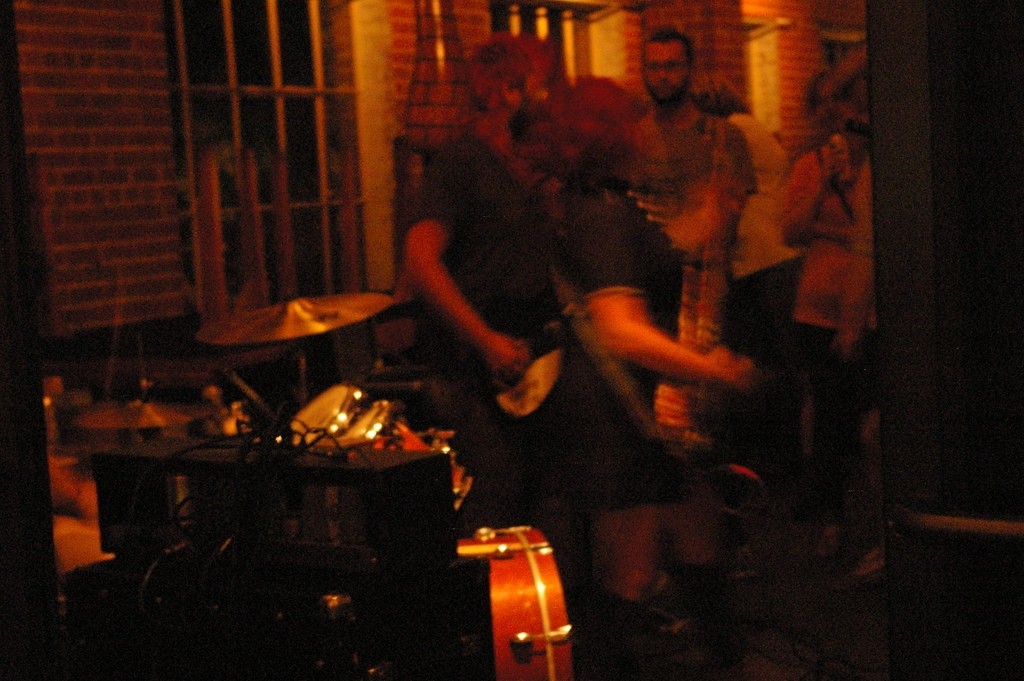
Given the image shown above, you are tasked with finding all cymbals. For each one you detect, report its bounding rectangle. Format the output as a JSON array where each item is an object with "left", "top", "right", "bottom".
[
  {"left": 74, "top": 402, "right": 206, "bottom": 434},
  {"left": 194, "top": 292, "right": 393, "bottom": 346}
]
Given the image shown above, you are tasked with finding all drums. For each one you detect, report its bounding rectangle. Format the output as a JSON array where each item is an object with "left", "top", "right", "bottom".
[
  {"left": 335, "top": 398, "right": 475, "bottom": 514},
  {"left": 453, "top": 524, "right": 575, "bottom": 681},
  {"left": 285, "top": 385, "right": 372, "bottom": 450}
]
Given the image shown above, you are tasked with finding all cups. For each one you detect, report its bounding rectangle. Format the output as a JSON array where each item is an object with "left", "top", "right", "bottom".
[{"left": 830, "top": 130, "right": 852, "bottom": 189}]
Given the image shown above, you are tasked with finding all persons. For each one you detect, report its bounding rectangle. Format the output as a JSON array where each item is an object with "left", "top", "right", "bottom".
[{"left": 402, "top": 20, "right": 874, "bottom": 681}]
